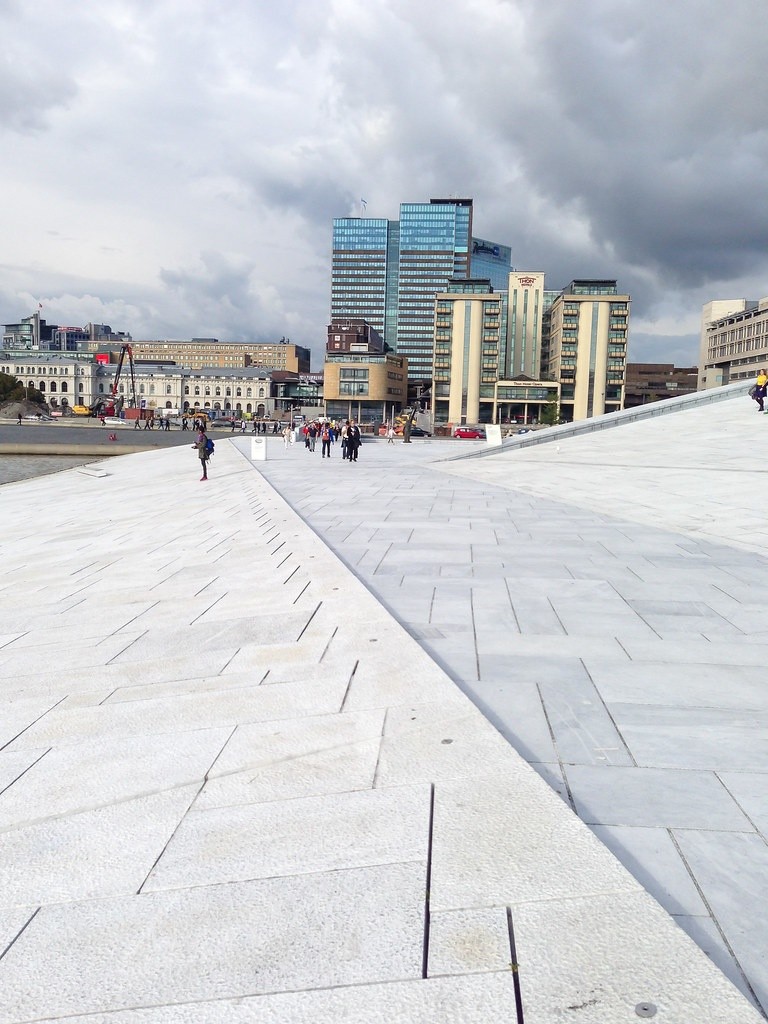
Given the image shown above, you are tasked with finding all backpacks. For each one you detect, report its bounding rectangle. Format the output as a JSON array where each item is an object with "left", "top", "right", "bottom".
[{"left": 204, "top": 438, "right": 214, "bottom": 455}]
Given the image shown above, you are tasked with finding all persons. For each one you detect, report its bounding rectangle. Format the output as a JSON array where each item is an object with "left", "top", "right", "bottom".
[
  {"left": 17, "top": 411, "right": 22, "bottom": 426},
  {"left": 231, "top": 417, "right": 362, "bottom": 462},
  {"left": 134, "top": 415, "right": 207, "bottom": 432},
  {"left": 387, "top": 427, "right": 397, "bottom": 444},
  {"left": 752, "top": 370, "right": 768, "bottom": 415},
  {"left": 191, "top": 426, "right": 209, "bottom": 481},
  {"left": 100, "top": 414, "right": 105, "bottom": 426}
]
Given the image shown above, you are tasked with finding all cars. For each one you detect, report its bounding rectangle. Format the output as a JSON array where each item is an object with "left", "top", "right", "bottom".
[
  {"left": 409, "top": 427, "right": 431, "bottom": 438},
  {"left": 454, "top": 428, "right": 486, "bottom": 439}
]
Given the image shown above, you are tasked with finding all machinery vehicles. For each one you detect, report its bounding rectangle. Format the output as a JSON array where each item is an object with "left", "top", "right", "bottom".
[
  {"left": 395, "top": 414, "right": 417, "bottom": 427},
  {"left": 71, "top": 343, "right": 137, "bottom": 418}
]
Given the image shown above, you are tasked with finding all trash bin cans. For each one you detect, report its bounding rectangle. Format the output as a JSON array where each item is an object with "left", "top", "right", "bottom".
[
  {"left": 250, "top": 437, "right": 267, "bottom": 461},
  {"left": 291, "top": 430, "right": 297, "bottom": 442}
]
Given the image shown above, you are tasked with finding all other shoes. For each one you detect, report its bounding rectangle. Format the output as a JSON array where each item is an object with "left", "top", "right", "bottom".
[
  {"left": 759, "top": 406, "right": 764, "bottom": 411},
  {"left": 200, "top": 477, "right": 208, "bottom": 481},
  {"left": 313, "top": 450, "right": 314, "bottom": 451}
]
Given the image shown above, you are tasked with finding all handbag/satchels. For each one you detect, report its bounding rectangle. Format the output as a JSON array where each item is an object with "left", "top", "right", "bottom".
[{"left": 322, "top": 436, "right": 330, "bottom": 441}]
